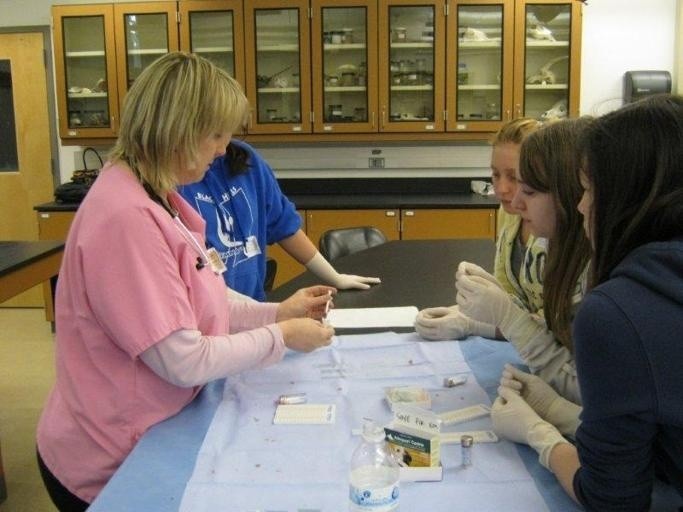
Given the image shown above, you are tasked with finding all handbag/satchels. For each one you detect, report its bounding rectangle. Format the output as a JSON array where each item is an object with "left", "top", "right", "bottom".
[{"left": 55, "top": 167, "right": 103, "bottom": 201}]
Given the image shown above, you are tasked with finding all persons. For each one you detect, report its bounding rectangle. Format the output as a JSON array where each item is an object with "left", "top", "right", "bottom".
[
  {"left": 454, "top": 115, "right": 592, "bottom": 407},
  {"left": 175, "top": 139, "right": 382, "bottom": 304},
  {"left": 33, "top": 53, "right": 336, "bottom": 511},
  {"left": 410, "top": 116, "right": 543, "bottom": 340},
  {"left": 490, "top": 94, "right": 683, "bottom": 509}
]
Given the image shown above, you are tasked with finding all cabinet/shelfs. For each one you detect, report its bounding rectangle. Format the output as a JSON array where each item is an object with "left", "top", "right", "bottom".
[
  {"left": 178, "top": 0, "right": 312, "bottom": 143},
  {"left": 306, "top": 208, "right": 498, "bottom": 253},
  {"left": 51, "top": 0, "right": 178, "bottom": 146},
  {"left": 445, "top": 0, "right": 583, "bottom": 142},
  {"left": 37, "top": 207, "right": 305, "bottom": 330},
  {"left": 310, "top": 0, "right": 445, "bottom": 142}
]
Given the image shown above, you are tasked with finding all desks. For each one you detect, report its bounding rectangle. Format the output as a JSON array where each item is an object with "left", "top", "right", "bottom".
[
  {"left": 83, "top": 237, "right": 683, "bottom": 512},
  {"left": 0, "top": 240, "right": 65, "bottom": 313}
]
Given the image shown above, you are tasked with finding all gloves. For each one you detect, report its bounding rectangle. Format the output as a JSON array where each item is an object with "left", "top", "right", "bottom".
[
  {"left": 414, "top": 261, "right": 583, "bottom": 472},
  {"left": 305, "top": 250, "right": 381, "bottom": 288}
]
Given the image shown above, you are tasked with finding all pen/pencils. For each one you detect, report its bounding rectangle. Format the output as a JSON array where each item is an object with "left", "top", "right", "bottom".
[{"left": 324, "top": 290, "right": 332, "bottom": 317}]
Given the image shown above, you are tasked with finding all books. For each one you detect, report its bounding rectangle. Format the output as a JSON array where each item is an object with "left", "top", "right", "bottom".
[{"left": 325, "top": 306, "right": 421, "bottom": 332}]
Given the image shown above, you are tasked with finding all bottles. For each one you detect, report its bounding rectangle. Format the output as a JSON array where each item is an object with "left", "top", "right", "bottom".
[
  {"left": 347, "top": 420, "right": 400, "bottom": 512},
  {"left": 268, "top": 27, "right": 498, "bottom": 121},
  {"left": 70, "top": 110, "right": 82, "bottom": 127},
  {"left": 460, "top": 435, "right": 475, "bottom": 468},
  {"left": 279, "top": 393, "right": 307, "bottom": 405},
  {"left": 368, "top": 150, "right": 385, "bottom": 168},
  {"left": 444, "top": 375, "right": 467, "bottom": 387}
]
